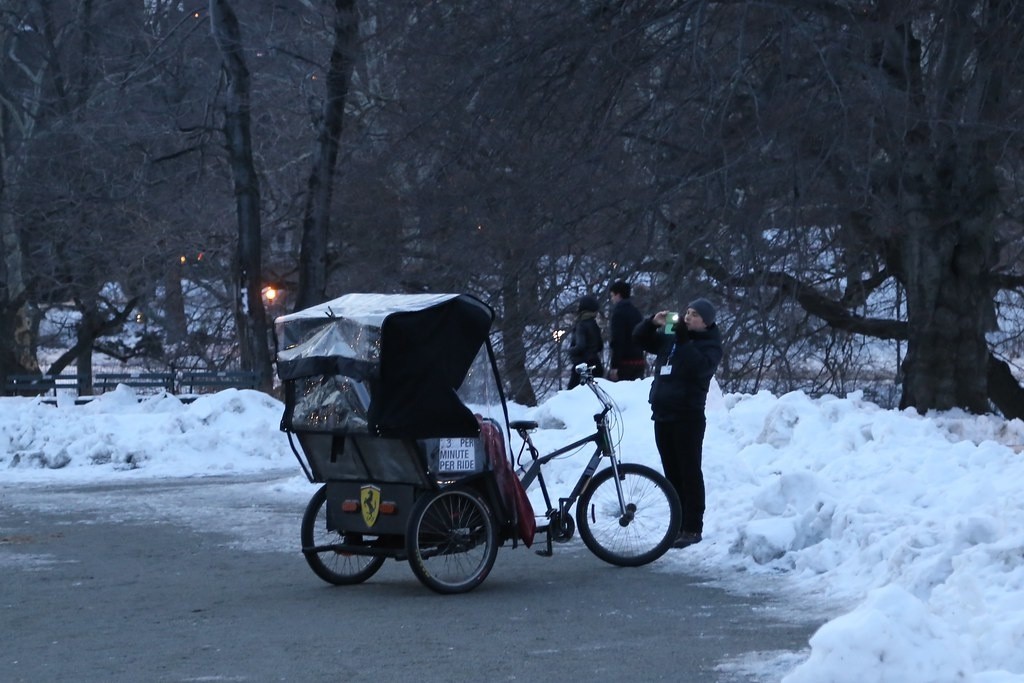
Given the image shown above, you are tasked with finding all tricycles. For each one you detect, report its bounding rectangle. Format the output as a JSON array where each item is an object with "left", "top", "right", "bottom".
[{"left": 271, "top": 291, "right": 683, "bottom": 594}]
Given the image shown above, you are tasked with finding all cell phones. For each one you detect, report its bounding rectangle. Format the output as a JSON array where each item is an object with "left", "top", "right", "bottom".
[{"left": 666, "top": 312, "right": 679, "bottom": 324}]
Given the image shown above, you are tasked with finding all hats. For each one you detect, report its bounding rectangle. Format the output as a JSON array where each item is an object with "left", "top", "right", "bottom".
[
  {"left": 610, "top": 282, "right": 631, "bottom": 299},
  {"left": 687, "top": 299, "right": 716, "bottom": 327},
  {"left": 579, "top": 296, "right": 600, "bottom": 313}
]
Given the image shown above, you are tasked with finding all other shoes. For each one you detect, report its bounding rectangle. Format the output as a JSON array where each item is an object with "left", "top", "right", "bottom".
[{"left": 673, "top": 532, "right": 702, "bottom": 548}]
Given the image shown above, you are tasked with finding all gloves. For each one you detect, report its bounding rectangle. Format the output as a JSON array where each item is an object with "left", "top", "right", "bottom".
[{"left": 671, "top": 316, "right": 688, "bottom": 341}]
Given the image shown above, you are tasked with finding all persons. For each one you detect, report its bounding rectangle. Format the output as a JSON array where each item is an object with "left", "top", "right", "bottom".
[
  {"left": 632, "top": 298, "right": 721, "bottom": 549},
  {"left": 606, "top": 281, "right": 645, "bottom": 383},
  {"left": 566, "top": 295, "right": 604, "bottom": 390}
]
